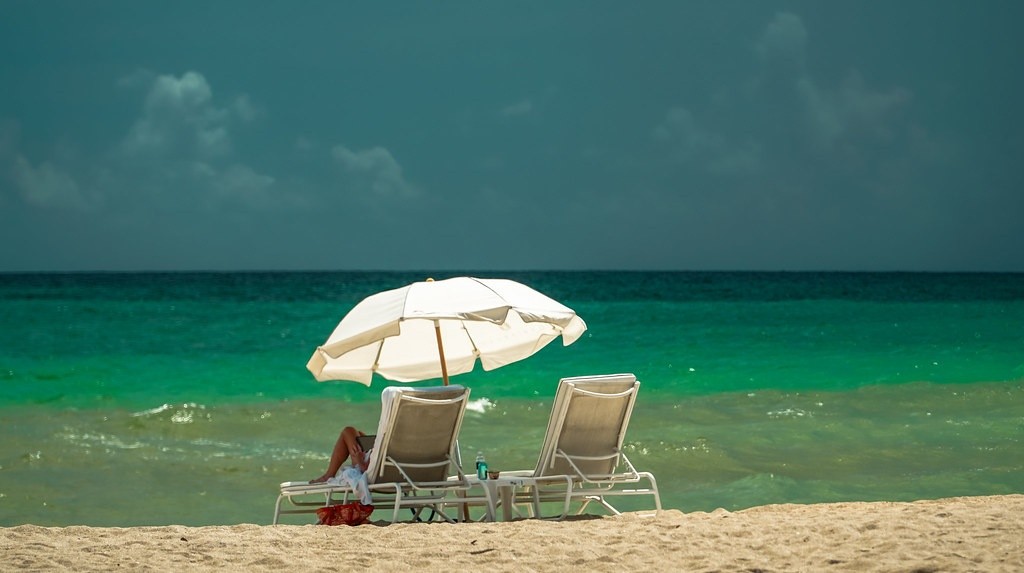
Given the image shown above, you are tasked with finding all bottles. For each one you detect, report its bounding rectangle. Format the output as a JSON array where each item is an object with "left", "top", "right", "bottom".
[
  {"left": 476, "top": 451, "right": 483, "bottom": 477},
  {"left": 478, "top": 456, "right": 488, "bottom": 480}
]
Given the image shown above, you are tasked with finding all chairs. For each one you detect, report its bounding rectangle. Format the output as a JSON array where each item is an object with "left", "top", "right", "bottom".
[
  {"left": 434, "top": 373, "right": 661, "bottom": 517},
  {"left": 273, "top": 383, "right": 497, "bottom": 526}
]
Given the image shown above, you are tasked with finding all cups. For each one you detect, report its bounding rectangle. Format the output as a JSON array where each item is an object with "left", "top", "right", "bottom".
[{"left": 489, "top": 471, "right": 500, "bottom": 479}]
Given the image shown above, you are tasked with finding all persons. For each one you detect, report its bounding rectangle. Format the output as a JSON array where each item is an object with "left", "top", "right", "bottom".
[{"left": 309, "top": 426, "right": 378, "bottom": 484}]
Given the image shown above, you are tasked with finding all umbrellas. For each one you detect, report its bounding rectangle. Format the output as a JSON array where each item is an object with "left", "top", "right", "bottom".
[{"left": 306, "top": 275, "right": 588, "bottom": 520}]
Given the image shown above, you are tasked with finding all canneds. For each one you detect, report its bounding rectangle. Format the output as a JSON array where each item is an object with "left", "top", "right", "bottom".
[{"left": 478, "top": 462, "right": 487, "bottom": 480}]
